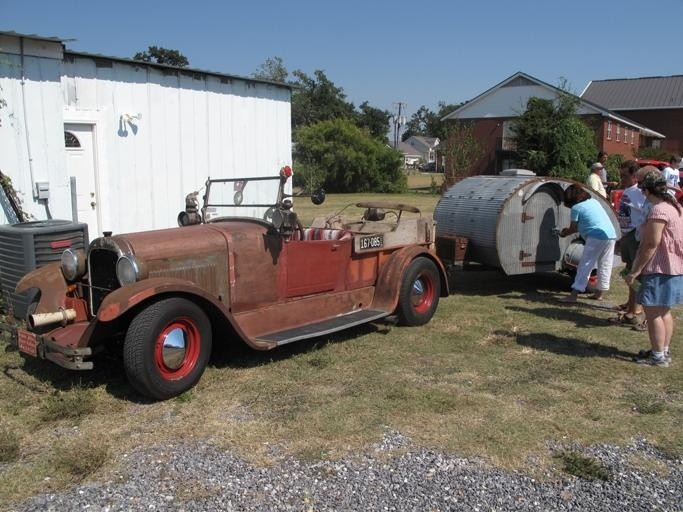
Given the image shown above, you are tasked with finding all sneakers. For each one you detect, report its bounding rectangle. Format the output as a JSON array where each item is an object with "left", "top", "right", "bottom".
[
  {"left": 632, "top": 321, "right": 649, "bottom": 333},
  {"left": 613, "top": 304, "right": 632, "bottom": 312},
  {"left": 609, "top": 313, "right": 639, "bottom": 328},
  {"left": 636, "top": 356, "right": 670, "bottom": 368},
  {"left": 640, "top": 347, "right": 674, "bottom": 364}
]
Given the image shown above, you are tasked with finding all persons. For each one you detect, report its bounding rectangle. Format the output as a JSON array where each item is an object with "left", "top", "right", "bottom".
[
  {"left": 624, "top": 170, "right": 683, "bottom": 366},
  {"left": 597, "top": 153, "right": 609, "bottom": 183},
  {"left": 607, "top": 166, "right": 663, "bottom": 330},
  {"left": 558, "top": 183, "right": 617, "bottom": 303},
  {"left": 614, "top": 160, "right": 647, "bottom": 312},
  {"left": 662, "top": 155, "right": 682, "bottom": 198},
  {"left": 585, "top": 163, "right": 618, "bottom": 201}
]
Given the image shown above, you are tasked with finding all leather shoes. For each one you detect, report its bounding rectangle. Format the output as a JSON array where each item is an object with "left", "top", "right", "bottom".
[
  {"left": 587, "top": 294, "right": 603, "bottom": 301},
  {"left": 556, "top": 296, "right": 579, "bottom": 303}
]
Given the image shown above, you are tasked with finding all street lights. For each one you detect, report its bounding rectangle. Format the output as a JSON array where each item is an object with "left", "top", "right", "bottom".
[
  {"left": 123, "top": 114, "right": 142, "bottom": 134},
  {"left": 393, "top": 114, "right": 406, "bottom": 149}
]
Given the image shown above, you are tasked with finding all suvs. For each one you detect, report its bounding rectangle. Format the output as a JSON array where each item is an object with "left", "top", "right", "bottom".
[{"left": 12, "top": 165, "right": 450, "bottom": 401}]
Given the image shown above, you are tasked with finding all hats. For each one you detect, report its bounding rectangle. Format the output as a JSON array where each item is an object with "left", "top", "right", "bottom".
[
  {"left": 636, "top": 172, "right": 667, "bottom": 189},
  {"left": 591, "top": 162, "right": 604, "bottom": 171}
]
